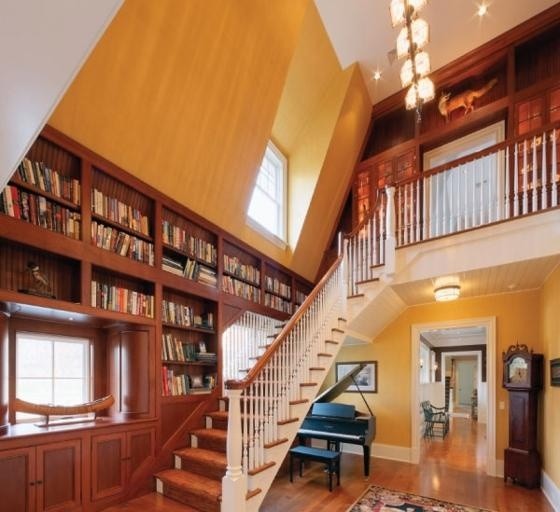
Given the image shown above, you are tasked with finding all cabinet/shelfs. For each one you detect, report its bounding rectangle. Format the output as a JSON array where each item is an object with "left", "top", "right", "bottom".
[
  {"left": 222, "top": 238, "right": 261, "bottom": 303},
  {"left": 160, "top": 204, "right": 219, "bottom": 288},
  {"left": 264, "top": 261, "right": 292, "bottom": 313},
  {"left": 90, "top": 166, "right": 155, "bottom": 268},
  {"left": 91, "top": 267, "right": 155, "bottom": 320},
  {"left": 163, "top": 285, "right": 218, "bottom": 396},
  {"left": 0, "top": 134, "right": 82, "bottom": 242},
  {"left": 1, "top": 236, "right": 81, "bottom": 305},
  {"left": 91, "top": 427, "right": 157, "bottom": 503},
  {"left": 296, "top": 281, "right": 315, "bottom": 317},
  {"left": 2, "top": 437, "right": 81, "bottom": 512}
]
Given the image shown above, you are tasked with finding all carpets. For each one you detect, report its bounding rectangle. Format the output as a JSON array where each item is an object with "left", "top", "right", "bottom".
[{"left": 345, "top": 485, "right": 492, "bottom": 512}]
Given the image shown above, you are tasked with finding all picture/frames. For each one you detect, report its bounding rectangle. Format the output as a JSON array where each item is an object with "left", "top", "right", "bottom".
[
  {"left": 550, "top": 358, "right": 560, "bottom": 387},
  {"left": 336, "top": 361, "right": 377, "bottom": 393}
]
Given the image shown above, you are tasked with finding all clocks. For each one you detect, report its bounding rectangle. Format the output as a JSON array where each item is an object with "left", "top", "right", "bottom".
[{"left": 502, "top": 344, "right": 543, "bottom": 489}]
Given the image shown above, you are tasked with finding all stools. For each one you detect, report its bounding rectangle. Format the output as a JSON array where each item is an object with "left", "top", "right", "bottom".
[{"left": 289, "top": 445, "right": 341, "bottom": 492}]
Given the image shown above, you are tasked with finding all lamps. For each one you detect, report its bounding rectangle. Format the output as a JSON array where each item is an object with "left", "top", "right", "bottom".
[
  {"left": 390, "top": 0, "right": 435, "bottom": 123},
  {"left": 434, "top": 285, "right": 461, "bottom": 302},
  {"left": 420, "top": 359, "right": 423, "bottom": 368}
]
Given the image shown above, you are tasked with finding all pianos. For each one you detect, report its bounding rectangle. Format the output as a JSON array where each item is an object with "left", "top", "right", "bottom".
[{"left": 296, "top": 363, "right": 376, "bottom": 479}]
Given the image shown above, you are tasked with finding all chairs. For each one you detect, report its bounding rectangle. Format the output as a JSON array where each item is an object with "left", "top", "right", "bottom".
[{"left": 422, "top": 401, "right": 448, "bottom": 440}]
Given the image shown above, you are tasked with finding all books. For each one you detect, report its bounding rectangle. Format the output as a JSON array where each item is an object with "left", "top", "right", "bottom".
[
  {"left": 161, "top": 367, "right": 218, "bottom": 396},
  {"left": 162, "top": 221, "right": 218, "bottom": 288},
  {"left": 264, "top": 276, "right": 307, "bottom": 315},
  {"left": 4, "top": 158, "right": 81, "bottom": 240},
  {"left": 91, "top": 189, "right": 155, "bottom": 266},
  {"left": 161, "top": 299, "right": 213, "bottom": 330},
  {"left": 91, "top": 281, "right": 154, "bottom": 318},
  {"left": 160, "top": 334, "right": 216, "bottom": 363},
  {"left": 222, "top": 255, "right": 260, "bottom": 304}
]
